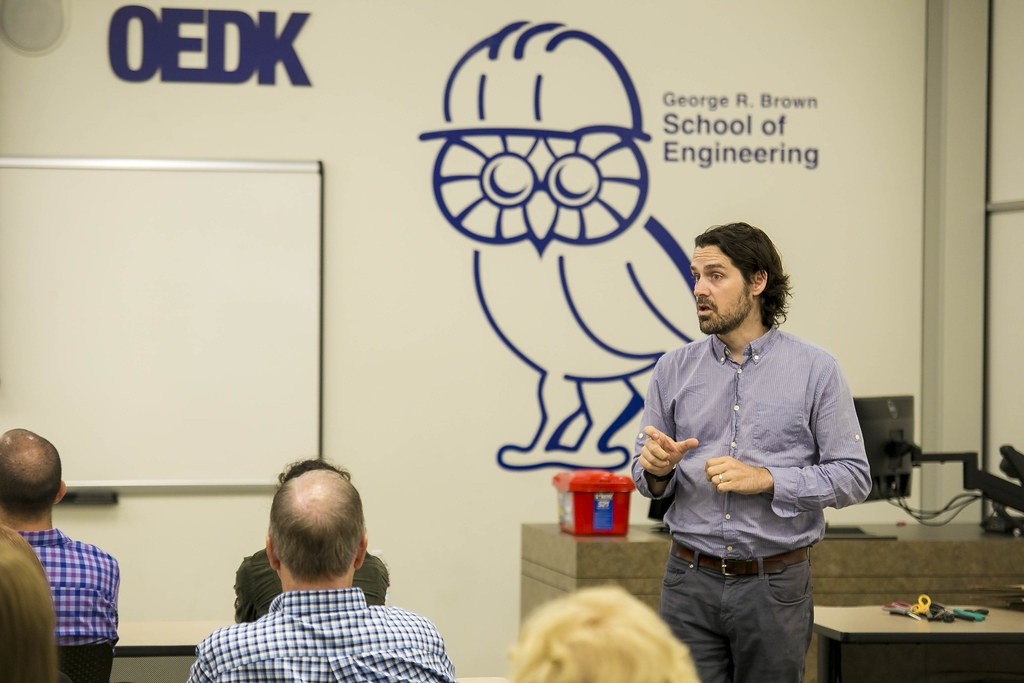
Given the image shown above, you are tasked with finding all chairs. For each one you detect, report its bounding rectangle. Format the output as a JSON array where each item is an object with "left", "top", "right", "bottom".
[{"left": 56, "top": 640, "right": 114, "bottom": 682}]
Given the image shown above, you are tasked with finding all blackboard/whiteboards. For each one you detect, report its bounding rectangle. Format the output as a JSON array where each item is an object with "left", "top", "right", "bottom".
[{"left": 1, "top": 154, "right": 326, "bottom": 492}]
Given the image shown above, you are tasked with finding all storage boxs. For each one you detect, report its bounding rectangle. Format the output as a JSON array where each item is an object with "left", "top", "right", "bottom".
[{"left": 553, "top": 468, "right": 637, "bottom": 536}]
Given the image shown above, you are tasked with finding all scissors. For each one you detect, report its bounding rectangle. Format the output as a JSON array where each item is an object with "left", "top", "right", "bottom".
[{"left": 910, "top": 594, "right": 933, "bottom": 619}]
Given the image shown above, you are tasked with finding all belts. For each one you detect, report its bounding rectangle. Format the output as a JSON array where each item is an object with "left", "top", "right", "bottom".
[{"left": 668, "top": 541, "right": 809, "bottom": 575}]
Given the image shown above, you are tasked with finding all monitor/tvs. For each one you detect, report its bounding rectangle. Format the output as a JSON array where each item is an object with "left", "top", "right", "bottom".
[{"left": 853, "top": 395, "right": 914, "bottom": 501}]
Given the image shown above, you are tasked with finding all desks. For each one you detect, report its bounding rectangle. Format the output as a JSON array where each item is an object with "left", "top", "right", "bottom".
[
  {"left": 809, "top": 605, "right": 1024, "bottom": 682},
  {"left": 517, "top": 521, "right": 1023, "bottom": 683}
]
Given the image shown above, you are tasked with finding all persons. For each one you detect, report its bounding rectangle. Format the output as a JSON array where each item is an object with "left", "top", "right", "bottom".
[
  {"left": 186, "top": 469, "right": 455, "bottom": 683},
  {"left": 509, "top": 586, "right": 698, "bottom": 683},
  {"left": 631, "top": 222, "right": 873, "bottom": 683},
  {"left": 0, "top": 525, "right": 72, "bottom": 683},
  {"left": 0, "top": 428, "right": 120, "bottom": 683},
  {"left": 233, "top": 460, "right": 390, "bottom": 623}
]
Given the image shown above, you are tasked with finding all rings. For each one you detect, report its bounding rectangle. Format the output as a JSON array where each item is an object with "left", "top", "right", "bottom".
[{"left": 719, "top": 474, "right": 722, "bottom": 483}]
